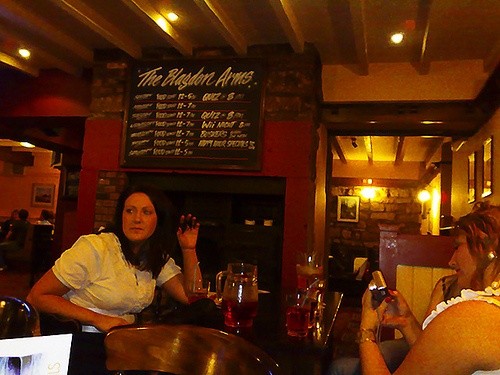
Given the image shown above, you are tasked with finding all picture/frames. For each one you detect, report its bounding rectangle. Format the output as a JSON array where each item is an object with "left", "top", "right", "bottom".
[
  {"left": 467, "top": 152, "right": 478, "bottom": 204},
  {"left": 30, "top": 183, "right": 56, "bottom": 208},
  {"left": 482, "top": 136, "right": 493, "bottom": 199},
  {"left": 338, "top": 195, "right": 362, "bottom": 223}
]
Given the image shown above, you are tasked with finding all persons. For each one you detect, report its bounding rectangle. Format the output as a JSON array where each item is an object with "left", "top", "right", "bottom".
[
  {"left": 0, "top": 209, "right": 31, "bottom": 271},
  {"left": 0, "top": 209, "right": 19, "bottom": 238},
  {"left": 18, "top": 182, "right": 203, "bottom": 375},
  {"left": 33, "top": 209, "right": 52, "bottom": 225},
  {"left": 355, "top": 200, "right": 500, "bottom": 375}
]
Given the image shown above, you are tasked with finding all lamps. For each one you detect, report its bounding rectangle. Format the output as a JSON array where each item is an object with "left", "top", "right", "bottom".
[{"left": 419, "top": 189, "right": 432, "bottom": 219}]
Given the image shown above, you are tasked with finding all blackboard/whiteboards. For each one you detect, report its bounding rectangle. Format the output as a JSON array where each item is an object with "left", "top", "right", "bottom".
[{"left": 125, "top": 56, "right": 264, "bottom": 170}]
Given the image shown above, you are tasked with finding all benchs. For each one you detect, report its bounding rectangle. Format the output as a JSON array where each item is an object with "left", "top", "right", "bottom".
[{"left": 378, "top": 231, "right": 461, "bottom": 342}]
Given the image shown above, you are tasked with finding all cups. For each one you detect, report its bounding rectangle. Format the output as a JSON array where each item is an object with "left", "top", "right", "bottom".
[
  {"left": 187, "top": 279, "right": 210, "bottom": 304},
  {"left": 286, "top": 296, "right": 310, "bottom": 336}
]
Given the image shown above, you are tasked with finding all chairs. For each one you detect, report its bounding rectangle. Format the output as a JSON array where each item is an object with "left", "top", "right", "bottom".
[
  {"left": 26, "top": 224, "right": 52, "bottom": 268},
  {"left": 102, "top": 324, "right": 286, "bottom": 375}
]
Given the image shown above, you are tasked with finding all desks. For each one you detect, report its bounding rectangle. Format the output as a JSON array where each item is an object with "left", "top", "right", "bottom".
[{"left": 136, "top": 284, "right": 343, "bottom": 374}]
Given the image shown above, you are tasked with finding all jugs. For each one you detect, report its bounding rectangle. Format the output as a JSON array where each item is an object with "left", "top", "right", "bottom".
[{"left": 216, "top": 263, "right": 258, "bottom": 327}]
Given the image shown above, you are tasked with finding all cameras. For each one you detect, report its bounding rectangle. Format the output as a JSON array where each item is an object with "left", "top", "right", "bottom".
[{"left": 368, "top": 270, "right": 389, "bottom": 304}]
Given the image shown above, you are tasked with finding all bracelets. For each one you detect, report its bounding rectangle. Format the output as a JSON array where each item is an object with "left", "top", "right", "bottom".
[{"left": 356, "top": 328, "right": 377, "bottom": 344}]
